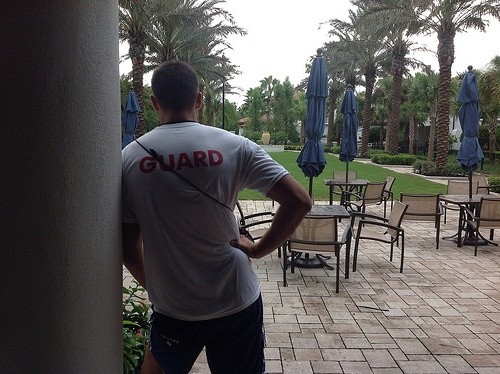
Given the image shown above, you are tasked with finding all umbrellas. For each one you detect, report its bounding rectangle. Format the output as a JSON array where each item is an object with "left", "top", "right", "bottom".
[
  {"left": 455, "top": 64, "right": 484, "bottom": 246},
  {"left": 340, "top": 85, "right": 358, "bottom": 218},
  {"left": 297, "top": 50, "right": 327, "bottom": 266},
  {"left": 123, "top": 88, "right": 140, "bottom": 149}
]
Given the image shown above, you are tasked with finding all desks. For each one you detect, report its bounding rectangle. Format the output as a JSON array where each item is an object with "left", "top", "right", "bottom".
[
  {"left": 323, "top": 179, "right": 368, "bottom": 220},
  {"left": 438, "top": 194, "right": 500, "bottom": 248},
  {"left": 286, "top": 205, "right": 351, "bottom": 270}
]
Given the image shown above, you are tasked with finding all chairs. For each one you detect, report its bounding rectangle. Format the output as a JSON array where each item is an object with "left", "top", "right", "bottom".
[
  {"left": 464, "top": 197, "right": 500, "bottom": 256},
  {"left": 236, "top": 201, "right": 281, "bottom": 258},
  {"left": 367, "top": 174, "right": 396, "bottom": 212},
  {"left": 329, "top": 171, "right": 359, "bottom": 205},
  {"left": 472, "top": 171, "right": 490, "bottom": 194},
  {"left": 396, "top": 192, "right": 445, "bottom": 249},
  {"left": 283, "top": 215, "right": 351, "bottom": 293},
  {"left": 339, "top": 181, "right": 387, "bottom": 226},
  {"left": 351, "top": 200, "right": 409, "bottom": 273},
  {"left": 444, "top": 179, "right": 471, "bottom": 224}
]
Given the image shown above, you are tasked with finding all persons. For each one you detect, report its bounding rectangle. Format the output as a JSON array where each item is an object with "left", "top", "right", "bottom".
[{"left": 122, "top": 61, "right": 313, "bottom": 374}]
